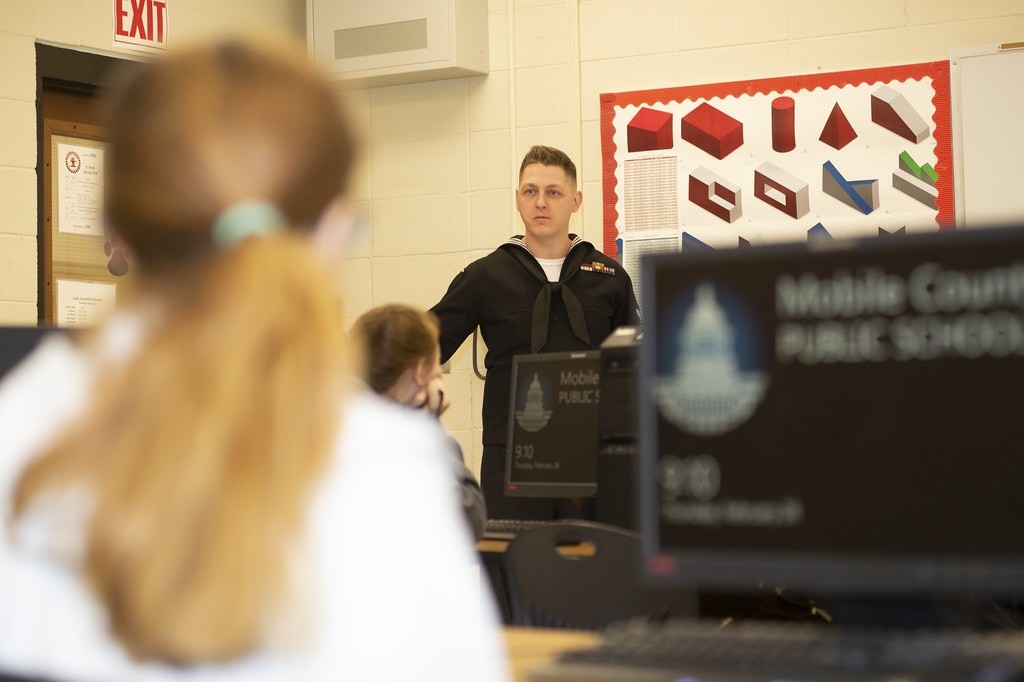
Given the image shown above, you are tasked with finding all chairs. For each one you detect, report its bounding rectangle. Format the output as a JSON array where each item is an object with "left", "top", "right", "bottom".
[{"left": 498, "top": 520, "right": 642, "bottom": 628}]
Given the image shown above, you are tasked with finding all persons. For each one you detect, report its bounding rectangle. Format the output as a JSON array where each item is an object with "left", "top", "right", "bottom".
[
  {"left": 1, "top": 34, "right": 509, "bottom": 682},
  {"left": 423, "top": 146, "right": 642, "bottom": 519},
  {"left": 343, "top": 303, "right": 451, "bottom": 424}
]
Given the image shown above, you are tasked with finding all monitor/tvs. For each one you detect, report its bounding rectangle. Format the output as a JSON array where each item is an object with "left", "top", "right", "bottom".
[
  {"left": 505, "top": 350, "right": 598, "bottom": 523},
  {"left": 646, "top": 219, "right": 1024, "bottom": 621}
]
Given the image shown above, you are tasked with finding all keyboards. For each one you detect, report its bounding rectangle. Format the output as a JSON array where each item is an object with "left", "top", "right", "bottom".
[
  {"left": 554, "top": 626, "right": 1024, "bottom": 682},
  {"left": 484, "top": 519, "right": 544, "bottom": 540}
]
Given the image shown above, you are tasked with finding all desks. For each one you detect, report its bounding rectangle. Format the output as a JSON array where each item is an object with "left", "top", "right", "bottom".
[{"left": 471, "top": 539, "right": 603, "bottom": 682}]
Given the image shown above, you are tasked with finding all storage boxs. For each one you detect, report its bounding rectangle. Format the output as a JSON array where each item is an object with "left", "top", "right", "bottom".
[{"left": 305, "top": 0, "right": 489, "bottom": 89}]
